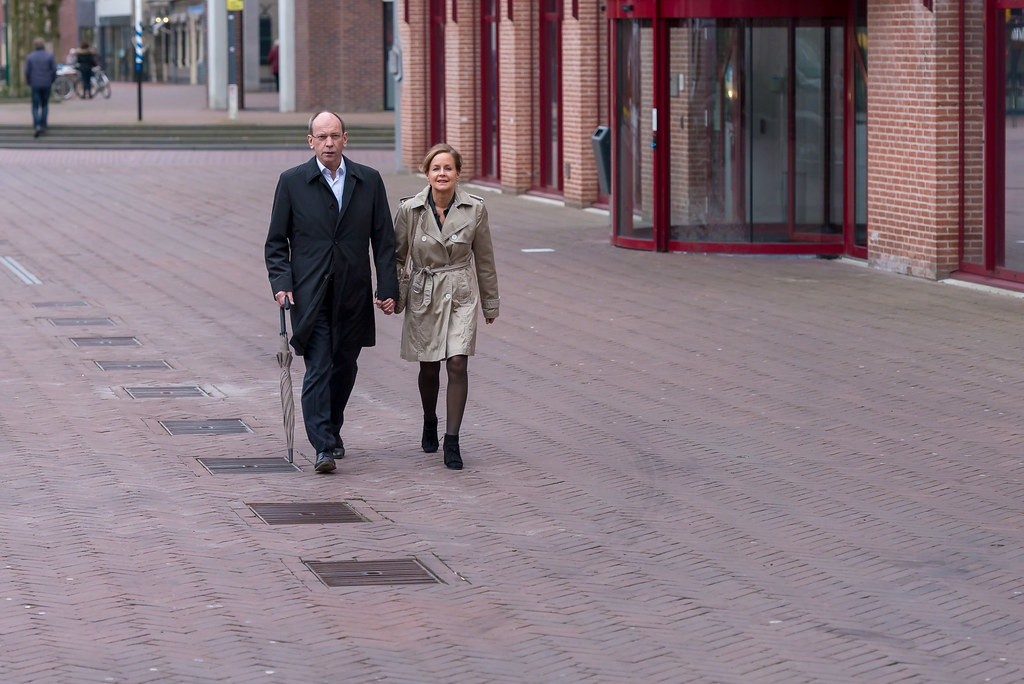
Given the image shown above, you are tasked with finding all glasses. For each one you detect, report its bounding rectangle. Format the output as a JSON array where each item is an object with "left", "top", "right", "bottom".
[{"left": 311, "top": 133, "right": 344, "bottom": 141}]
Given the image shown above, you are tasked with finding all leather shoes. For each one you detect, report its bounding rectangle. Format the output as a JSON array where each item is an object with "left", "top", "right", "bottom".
[
  {"left": 314, "top": 450, "right": 336, "bottom": 471},
  {"left": 332, "top": 448, "right": 345, "bottom": 459}
]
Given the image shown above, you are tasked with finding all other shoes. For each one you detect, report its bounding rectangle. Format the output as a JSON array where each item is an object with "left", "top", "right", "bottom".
[{"left": 34, "top": 125, "right": 42, "bottom": 138}]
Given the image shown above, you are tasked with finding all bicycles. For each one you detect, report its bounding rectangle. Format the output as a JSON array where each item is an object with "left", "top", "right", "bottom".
[{"left": 50, "top": 62, "right": 112, "bottom": 100}]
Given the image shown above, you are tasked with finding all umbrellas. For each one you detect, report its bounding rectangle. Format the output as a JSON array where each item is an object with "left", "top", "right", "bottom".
[{"left": 276, "top": 296, "right": 295, "bottom": 462}]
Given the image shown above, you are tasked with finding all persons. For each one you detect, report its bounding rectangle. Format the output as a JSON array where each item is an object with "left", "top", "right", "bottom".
[
  {"left": 267, "top": 39, "right": 279, "bottom": 93},
  {"left": 265, "top": 111, "right": 399, "bottom": 473},
  {"left": 66, "top": 40, "right": 98, "bottom": 98},
  {"left": 373, "top": 143, "right": 500, "bottom": 470},
  {"left": 24, "top": 37, "right": 58, "bottom": 137}
]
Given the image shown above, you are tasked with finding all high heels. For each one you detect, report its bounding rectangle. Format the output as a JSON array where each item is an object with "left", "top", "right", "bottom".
[
  {"left": 443, "top": 433, "right": 463, "bottom": 470},
  {"left": 422, "top": 414, "right": 439, "bottom": 452}
]
